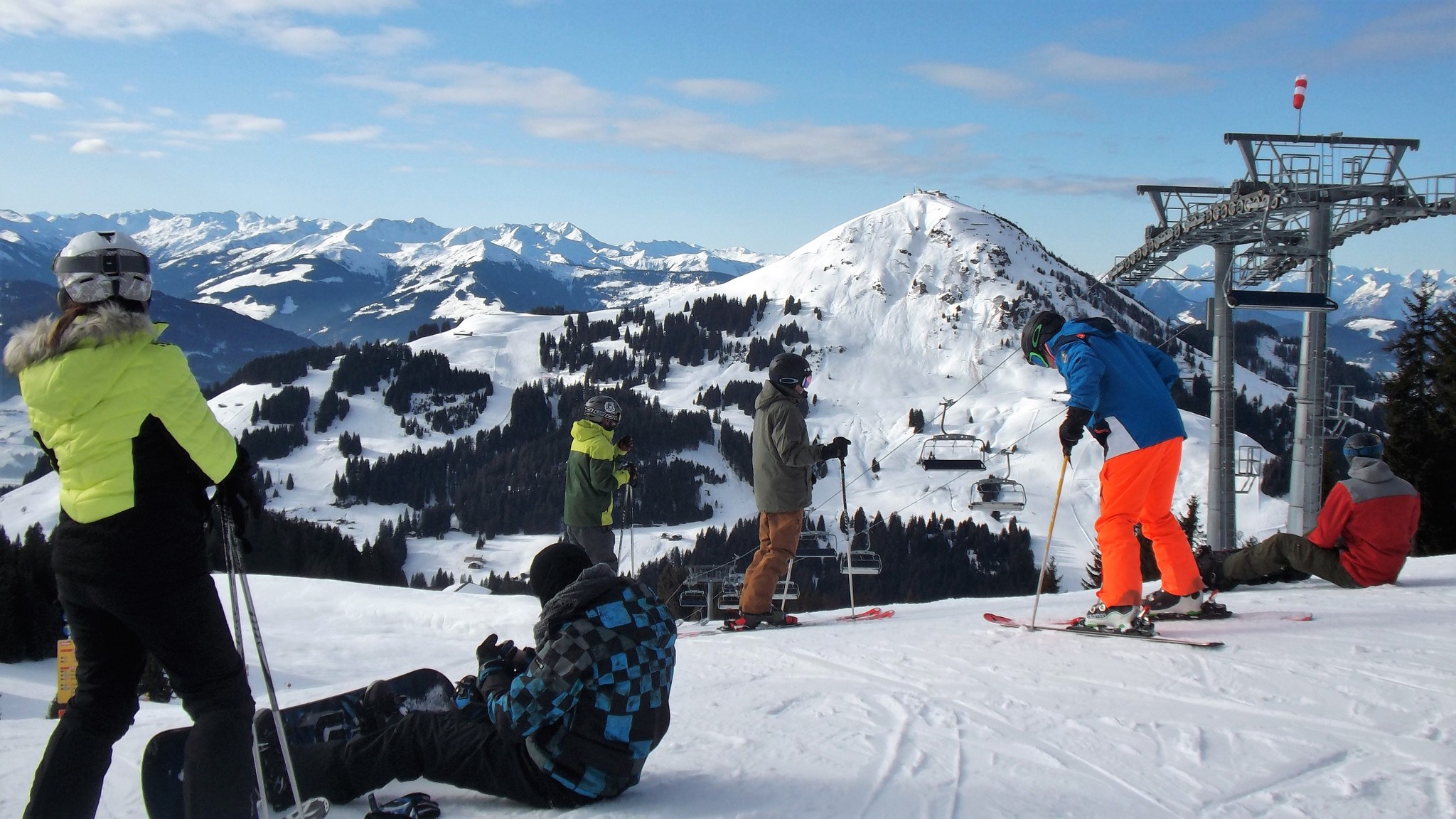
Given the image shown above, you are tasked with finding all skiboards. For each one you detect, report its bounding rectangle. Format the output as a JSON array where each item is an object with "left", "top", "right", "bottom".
[
  {"left": 983, "top": 588, "right": 1314, "bottom": 647},
  {"left": 677, "top": 607, "right": 895, "bottom": 639}
]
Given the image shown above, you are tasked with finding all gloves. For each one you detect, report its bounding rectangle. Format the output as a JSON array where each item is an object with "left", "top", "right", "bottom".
[
  {"left": 364, "top": 792, "right": 441, "bottom": 819},
  {"left": 823, "top": 439, "right": 848, "bottom": 459},
  {"left": 1059, "top": 416, "right": 1084, "bottom": 448},
  {"left": 476, "top": 634, "right": 518, "bottom": 667},
  {"left": 618, "top": 434, "right": 633, "bottom": 451},
  {"left": 212, "top": 445, "right": 260, "bottom": 521},
  {"left": 623, "top": 465, "right": 640, "bottom": 485}
]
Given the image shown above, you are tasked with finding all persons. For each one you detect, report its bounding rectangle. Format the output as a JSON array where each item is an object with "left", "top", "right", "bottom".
[
  {"left": 1020, "top": 311, "right": 1203, "bottom": 633},
  {"left": 564, "top": 395, "right": 639, "bottom": 573},
  {"left": 739, "top": 353, "right": 852, "bottom": 627},
  {"left": 252, "top": 543, "right": 677, "bottom": 810},
  {"left": 3, "top": 231, "right": 259, "bottom": 819},
  {"left": 1193, "top": 433, "right": 1421, "bottom": 589}
]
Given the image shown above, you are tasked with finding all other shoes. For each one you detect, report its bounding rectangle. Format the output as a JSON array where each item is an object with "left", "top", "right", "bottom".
[
  {"left": 252, "top": 708, "right": 291, "bottom": 772},
  {"left": 1140, "top": 588, "right": 1203, "bottom": 615},
  {"left": 1194, "top": 546, "right": 1233, "bottom": 590},
  {"left": 1086, "top": 602, "right": 1139, "bottom": 625},
  {"left": 364, "top": 680, "right": 405, "bottom": 732},
  {"left": 742, "top": 608, "right": 786, "bottom": 626}
]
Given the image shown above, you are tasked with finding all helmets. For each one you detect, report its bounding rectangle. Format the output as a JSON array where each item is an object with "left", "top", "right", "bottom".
[
  {"left": 1021, "top": 311, "right": 1066, "bottom": 368},
  {"left": 52, "top": 230, "right": 152, "bottom": 313},
  {"left": 584, "top": 395, "right": 622, "bottom": 422},
  {"left": 1343, "top": 433, "right": 1384, "bottom": 464},
  {"left": 769, "top": 353, "right": 812, "bottom": 398}
]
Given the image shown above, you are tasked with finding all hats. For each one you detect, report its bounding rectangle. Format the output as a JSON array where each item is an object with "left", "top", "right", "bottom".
[{"left": 530, "top": 543, "right": 593, "bottom": 611}]
